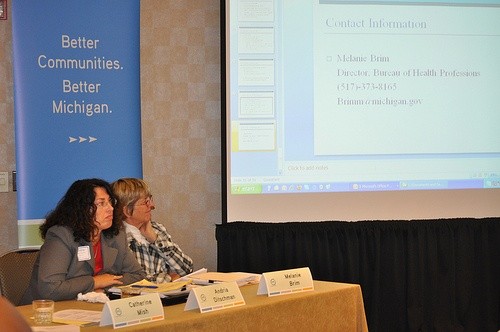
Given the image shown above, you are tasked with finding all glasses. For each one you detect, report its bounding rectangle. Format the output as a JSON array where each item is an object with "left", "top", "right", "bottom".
[
  {"left": 128, "top": 195, "right": 153, "bottom": 206},
  {"left": 94, "top": 198, "right": 117, "bottom": 207}
]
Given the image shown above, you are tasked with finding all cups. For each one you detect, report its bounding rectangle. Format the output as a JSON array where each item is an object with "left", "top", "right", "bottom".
[{"left": 32, "top": 300, "right": 54, "bottom": 325}]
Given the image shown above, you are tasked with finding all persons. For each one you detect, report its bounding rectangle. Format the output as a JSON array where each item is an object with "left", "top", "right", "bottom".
[
  {"left": 110, "top": 179, "right": 193, "bottom": 286},
  {"left": 18, "top": 178, "right": 147, "bottom": 306}
]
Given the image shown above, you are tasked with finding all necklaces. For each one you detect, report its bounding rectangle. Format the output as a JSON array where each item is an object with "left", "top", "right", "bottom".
[{"left": 94, "top": 244, "right": 98, "bottom": 259}]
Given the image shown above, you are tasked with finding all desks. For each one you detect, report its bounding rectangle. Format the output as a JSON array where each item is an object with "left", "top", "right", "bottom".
[{"left": 14, "top": 274, "right": 369, "bottom": 332}]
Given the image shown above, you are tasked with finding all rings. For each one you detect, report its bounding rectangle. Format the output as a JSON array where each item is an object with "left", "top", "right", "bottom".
[{"left": 114, "top": 276, "right": 116, "bottom": 279}]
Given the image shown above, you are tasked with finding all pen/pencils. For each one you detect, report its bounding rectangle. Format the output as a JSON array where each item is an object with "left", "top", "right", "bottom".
[{"left": 130, "top": 284, "right": 158, "bottom": 289}]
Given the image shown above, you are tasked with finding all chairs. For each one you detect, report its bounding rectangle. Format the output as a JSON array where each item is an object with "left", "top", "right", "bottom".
[{"left": 0, "top": 246, "right": 40, "bottom": 305}]
